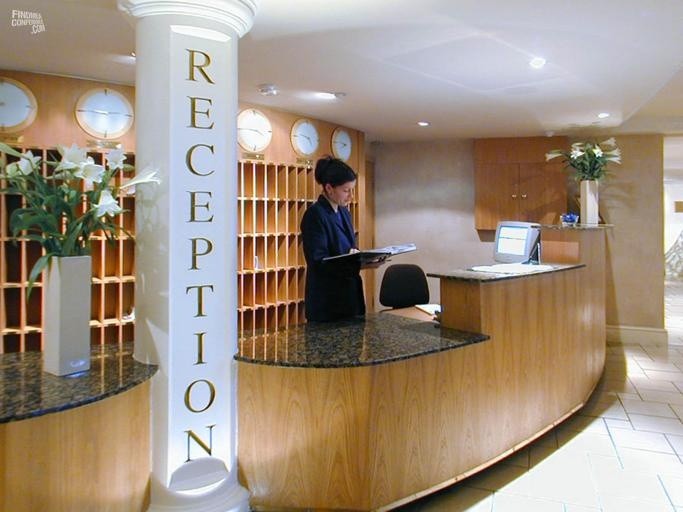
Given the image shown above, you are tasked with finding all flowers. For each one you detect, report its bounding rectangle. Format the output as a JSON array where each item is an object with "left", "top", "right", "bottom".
[
  {"left": 0, "top": 144, "right": 165, "bottom": 309},
  {"left": 543, "top": 137, "right": 622, "bottom": 184}
]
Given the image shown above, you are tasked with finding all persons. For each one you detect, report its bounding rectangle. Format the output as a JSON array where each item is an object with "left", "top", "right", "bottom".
[{"left": 300, "top": 157, "right": 390, "bottom": 322}]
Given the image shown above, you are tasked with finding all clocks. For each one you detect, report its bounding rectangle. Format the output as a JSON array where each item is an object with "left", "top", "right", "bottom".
[
  {"left": 329, "top": 123, "right": 353, "bottom": 167},
  {"left": 0, "top": 73, "right": 39, "bottom": 138},
  {"left": 289, "top": 115, "right": 322, "bottom": 159},
  {"left": 73, "top": 85, "right": 135, "bottom": 145},
  {"left": 235, "top": 104, "right": 276, "bottom": 155}
]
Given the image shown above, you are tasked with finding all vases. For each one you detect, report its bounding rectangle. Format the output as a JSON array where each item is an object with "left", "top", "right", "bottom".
[
  {"left": 577, "top": 179, "right": 601, "bottom": 231},
  {"left": 39, "top": 254, "right": 94, "bottom": 379}
]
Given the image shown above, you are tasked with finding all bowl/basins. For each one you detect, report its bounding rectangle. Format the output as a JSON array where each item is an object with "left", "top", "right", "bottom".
[{"left": 560, "top": 216, "right": 580, "bottom": 225}]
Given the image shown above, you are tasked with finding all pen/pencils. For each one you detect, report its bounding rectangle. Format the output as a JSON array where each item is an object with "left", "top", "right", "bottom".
[{"left": 385, "top": 259, "right": 392, "bottom": 261}]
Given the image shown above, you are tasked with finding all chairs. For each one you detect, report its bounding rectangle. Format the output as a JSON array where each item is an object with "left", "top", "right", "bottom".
[{"left": 375, "top": 263, "right": 430, "bottom": 315}]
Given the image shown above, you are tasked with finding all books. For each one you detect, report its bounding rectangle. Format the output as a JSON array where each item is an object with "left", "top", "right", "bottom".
[{"left": 323, "top": 243, "right": 418, "bottom": 264}]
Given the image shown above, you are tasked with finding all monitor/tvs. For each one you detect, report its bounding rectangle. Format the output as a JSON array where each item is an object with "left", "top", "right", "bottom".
[{"left": 495, "top": 222, "right": 531, "bottom": 263}]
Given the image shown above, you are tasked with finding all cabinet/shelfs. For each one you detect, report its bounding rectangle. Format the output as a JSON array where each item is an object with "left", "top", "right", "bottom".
[
  {"left": 0, "top": 144, "right": 358, "bottom": 346},
  {"left": 469, "top": 137, "right": 571, "bottom": 236}
]
